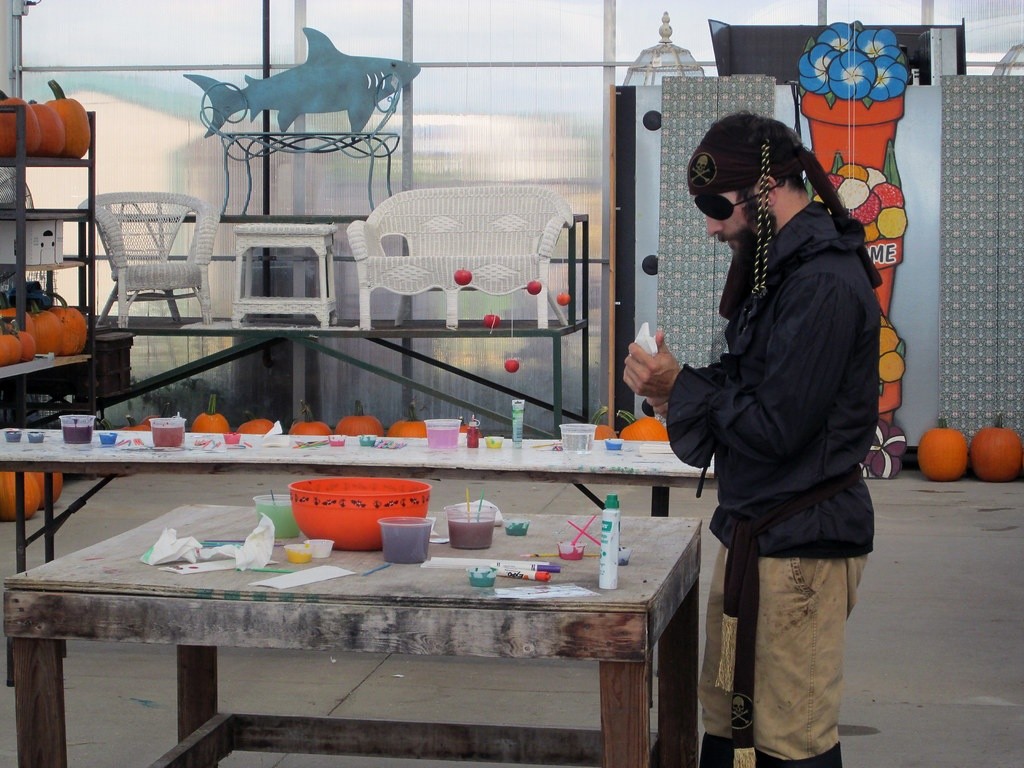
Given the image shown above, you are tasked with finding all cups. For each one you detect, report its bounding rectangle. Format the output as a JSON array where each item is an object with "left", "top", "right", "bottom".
[
  {"left": 503, "top": 519, "right": 531, "bottom": 536},
  {"left": 444, "top": 504, "right": 499, "bottom": 549},
  {"left": 466, "top": 566, "right": 497, "bottom": 587},
  {"left": 283, "top": 542, "right": 314, "bottom": 562},
  {"left": 253, "top": 494, "right": 301, "bottom": 538},
  {"left": 559, "top": 424, "right": 597, "bottom": 454},
  {"left": 424, "top": 419, "right": 462, "bottom": 449},
  {"left": 484, "top": 436, "right": 504, "bottom": 448},
  {"left": 557, "top": 540, "right": 587, "bottom": 560},
  {"left": 223, "top": 433, "right": 242, "bottom": 444},
  {"left": 304, "top": 539, "right": 334, "bottom": 558},
  {"left": 328, "top": 434, "right": 347, "bottom": 446},
  {"left": 149, "top": 419, "right": 184, "bottom": 447},
  {"left": 376, "top": 516, "right": 434, "bottom": 563},
  {"left": 59, "top": 415, "right": 96, "bottom": 444},
  {"left": 358, "top": 435, "right": 377, "bottom": 446},
  {"left": 604, "top": 438, "right": 624, "bottom": 451},
  {"left": 27, "top": 431, "right": 45, "bottom": 443},
  {"left": 619, "top": 546, "right": 633, "bottom": 565},
  {"left": 99, "top": 432, "right": 118, "bottom": 445},
  {"left": 5, "top": 430, "right": 22, "bottom": 442}
]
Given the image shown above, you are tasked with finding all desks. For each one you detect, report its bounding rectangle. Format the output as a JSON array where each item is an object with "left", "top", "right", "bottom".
[
  {"left": 231, "top": 223, "right": 338, "bottom": 329},
  {"left": 0, "top": 427, "right": 718, "bottom": 573}
]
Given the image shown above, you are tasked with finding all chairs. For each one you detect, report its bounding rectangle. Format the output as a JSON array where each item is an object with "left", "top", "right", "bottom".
[{"left": 77, "top": 192, "right": 221, "bottom": 328}]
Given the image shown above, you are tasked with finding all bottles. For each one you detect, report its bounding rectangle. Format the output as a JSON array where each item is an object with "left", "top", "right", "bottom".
[
  {"left": 599, "top": 494, "right": 620, "bottom": 590},
  {"left": 172, "top": 412, "right": 185, "bottom": 443},
  {"left": 467, "top": 415, "right": 479, "bottom": 448}
]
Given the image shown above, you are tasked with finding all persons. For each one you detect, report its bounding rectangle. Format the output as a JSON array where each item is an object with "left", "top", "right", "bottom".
[{"left": 623, "top": 109, "right": 883, "bottom": 768}]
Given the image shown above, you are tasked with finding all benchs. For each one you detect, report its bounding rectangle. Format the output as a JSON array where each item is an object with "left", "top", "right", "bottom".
[{"left": 346, "top": 185, "right": 574, "bottom": 331}]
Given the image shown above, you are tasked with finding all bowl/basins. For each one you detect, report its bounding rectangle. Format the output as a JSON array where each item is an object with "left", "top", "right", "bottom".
[{"left": 287, "top": 478, "right": 432, "bottom": 551}]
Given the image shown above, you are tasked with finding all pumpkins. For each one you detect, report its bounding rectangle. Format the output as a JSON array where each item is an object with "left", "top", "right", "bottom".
[
  {"left": 590, "top": 406, "right": 668, "bottom": 441},
  {"left": 0, "top": 80, "right": 91, "bottom": 159},
  {"left": 121, "top": 403, "right": 172, "bottom": 431},
  {"left": 918, "top": 417, "right": 967, "bottom": 483},
  {"left": 190, "top": 395, "right": 230, "bottom": 433},
  {"left": 0, "top": 289, "right": 86, "bottom": 366},
  {"left": 970, "top": 416, "right": 1022, "bottom": 482},
  {"left": 236, "top": 411, "right": 274, "bottom": 434},
  {"left": 387, "top": 403, "right": 482, "bottom": 437},
  {"left": 0, "top": 471, "right": 62, "bottom": 523},
  {"left": 288, "top": 400, "right": 333, "bottom": 435},
  {"left": 335, "top": 400, "right": 384, "bottom": 437}
]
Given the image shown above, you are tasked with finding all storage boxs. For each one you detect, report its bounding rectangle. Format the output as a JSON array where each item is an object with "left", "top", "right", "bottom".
[{"left": 0, "top": 219, "right": 63, "bottom": 265}]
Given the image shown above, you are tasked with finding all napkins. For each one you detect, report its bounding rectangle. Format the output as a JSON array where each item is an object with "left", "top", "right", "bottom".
[
  {"left": 245, "top": 564, "right": 358, "bottom": 589},
  {"left": 3, "top": 505, "right": 702, "bottom": 768},
  {"left": 638, "top": 443, "right": 674, "bottom": 454},
  {"left": 633, "top": 322, "right": 660, "bottom": 357}
]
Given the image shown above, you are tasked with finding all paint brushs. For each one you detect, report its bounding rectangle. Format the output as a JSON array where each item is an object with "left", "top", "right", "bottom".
[
  {"left": 244, "top": 441, "right": 252, "bottom": 447},
  {"left": 236, "top": 567, "right": 295, "bottom": 573},
  {"left": 200, "top": 539, "right": 285, "bottom": 548},
  {"left": 374, "top": 439, "right": 407, "bottom": 449},
  {"left": 115, "top": 437, "right": 144, "bottom": 446},
  {"left": 519, "top": 553, "right": 600, "bottom": 557},
  {"left": 532, "top": 443, "right": 562, "bottom": 449},
  {"left": 477, "top": 499, "right": 482, "bottom": 522},
  {"left": 270, "top": 489, "right": 276, "bottom": 504},
  {"left": 567, "top": 514, "right": 600, "bottom": 546},
  {"left": 194, "top": 438, "right": 222, "bottom": 450},
  {"left": 362, "top": 563, "right": 394, "bottom": 576},
  {"left": 466, "top": 488, "right": 471, "bottom": 522}
]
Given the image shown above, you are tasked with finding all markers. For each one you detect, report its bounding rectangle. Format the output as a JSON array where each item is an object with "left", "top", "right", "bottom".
[
  {"left": 490, "top": 562, "right": 561, "bottom": 573},
  {"left": 489, "top": 566, "right": 551, "bottom": 581}
]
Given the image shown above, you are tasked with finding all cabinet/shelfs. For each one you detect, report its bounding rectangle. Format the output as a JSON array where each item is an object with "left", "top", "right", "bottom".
[{"left": 0, "top": 104, "right": 95, "bottom": 428}]
